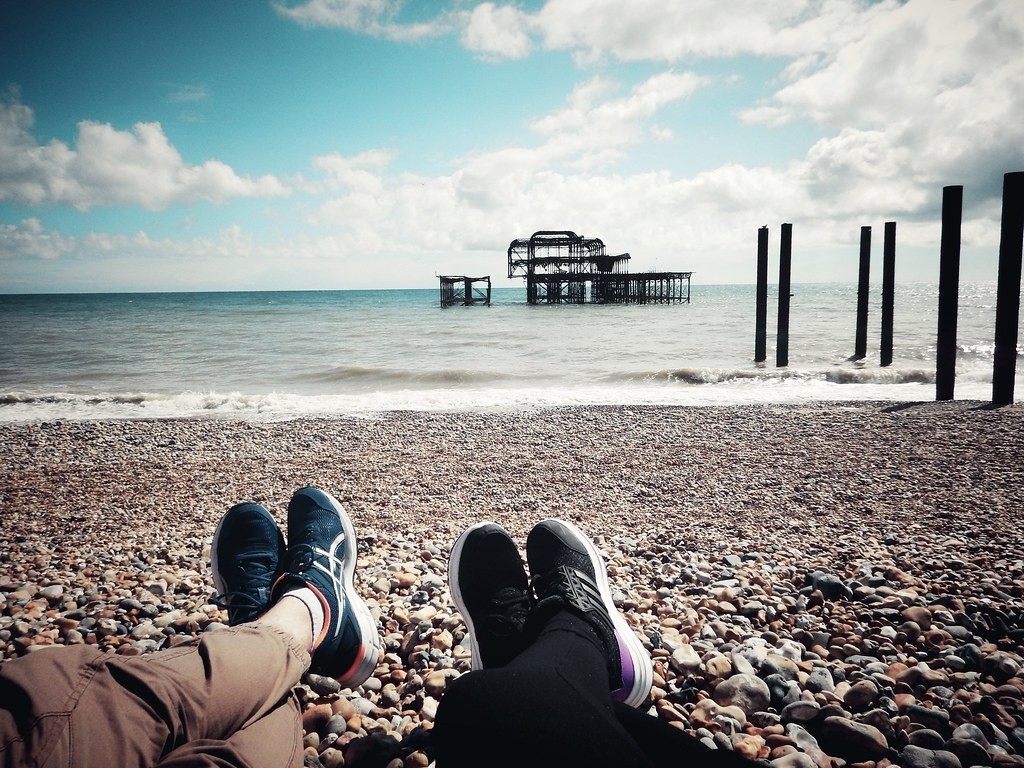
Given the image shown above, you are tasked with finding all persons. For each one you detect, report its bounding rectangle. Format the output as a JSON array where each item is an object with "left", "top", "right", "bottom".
[
  {"left": 432, "top": 519, "right": 760, "bottom": 768},
  {"left": 0, "top": 483, "right": 380, "bottom": 768}
]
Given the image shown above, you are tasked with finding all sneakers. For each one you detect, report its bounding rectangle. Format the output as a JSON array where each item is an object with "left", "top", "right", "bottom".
[
  {"left": 523, "top": 517, "right": 654, "bottom": 710},
  {"left": 449, "top": 520, "right": 538, "bottom": 673},
  {"left": 210, "top": 501, "right": 284, "bottom": 624},
  {"left": 269, "top": 486, "right": 380, "bottom": 687}
]
